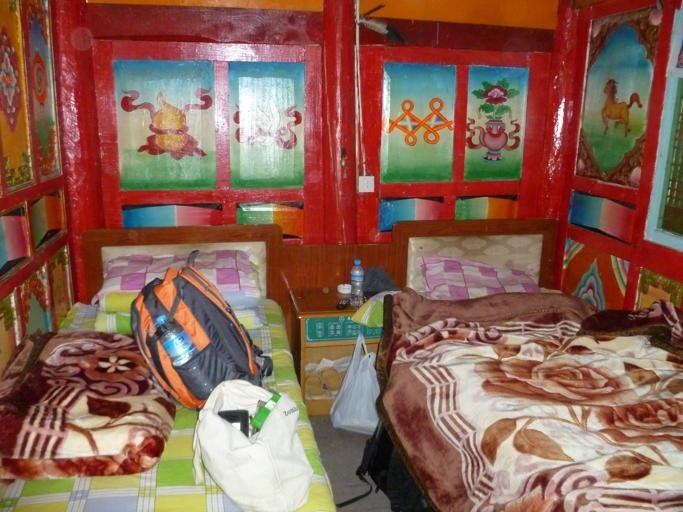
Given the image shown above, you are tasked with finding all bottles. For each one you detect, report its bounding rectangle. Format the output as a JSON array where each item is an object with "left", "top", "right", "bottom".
[
  {"left": 154, "top": 315, "right": 216, "bottom": 401},
  {"left": 349, "top": 258, "right": 365, "bottom": 308}
]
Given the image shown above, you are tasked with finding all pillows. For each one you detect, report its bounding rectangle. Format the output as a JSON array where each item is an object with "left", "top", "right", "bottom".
[
  {"left": 92, "top": 250, "right": 264, "bottom": 303},
  {"left": 420, "top": 256, "right": 540, "bottom": 300}
]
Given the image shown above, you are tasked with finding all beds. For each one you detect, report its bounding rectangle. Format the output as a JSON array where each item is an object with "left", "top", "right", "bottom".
[
  {"left": 0, "top": 225, "right": 338, "bottom": 512},
  {"left": 382, "top": 227, "right": 683, "bottom": 512}
]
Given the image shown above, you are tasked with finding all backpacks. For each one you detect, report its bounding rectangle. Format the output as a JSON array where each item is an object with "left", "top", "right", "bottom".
[{"left": 130, "top": 250, "right": 274, "bottom": 410}]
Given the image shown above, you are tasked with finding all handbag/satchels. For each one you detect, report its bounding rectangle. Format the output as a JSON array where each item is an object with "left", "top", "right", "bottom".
[{"left": 192, "top": 379, "right": 314, "bottom": 512}]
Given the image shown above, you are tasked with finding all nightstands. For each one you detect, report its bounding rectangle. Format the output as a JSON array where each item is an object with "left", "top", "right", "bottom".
[{"left": 286, "top": 285, "right": 386, "bottom": 421}]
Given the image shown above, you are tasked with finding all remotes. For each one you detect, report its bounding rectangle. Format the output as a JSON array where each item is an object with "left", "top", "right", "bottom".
[{"left": 336, "top": 298, "right": 350, "bottom": 309}]
